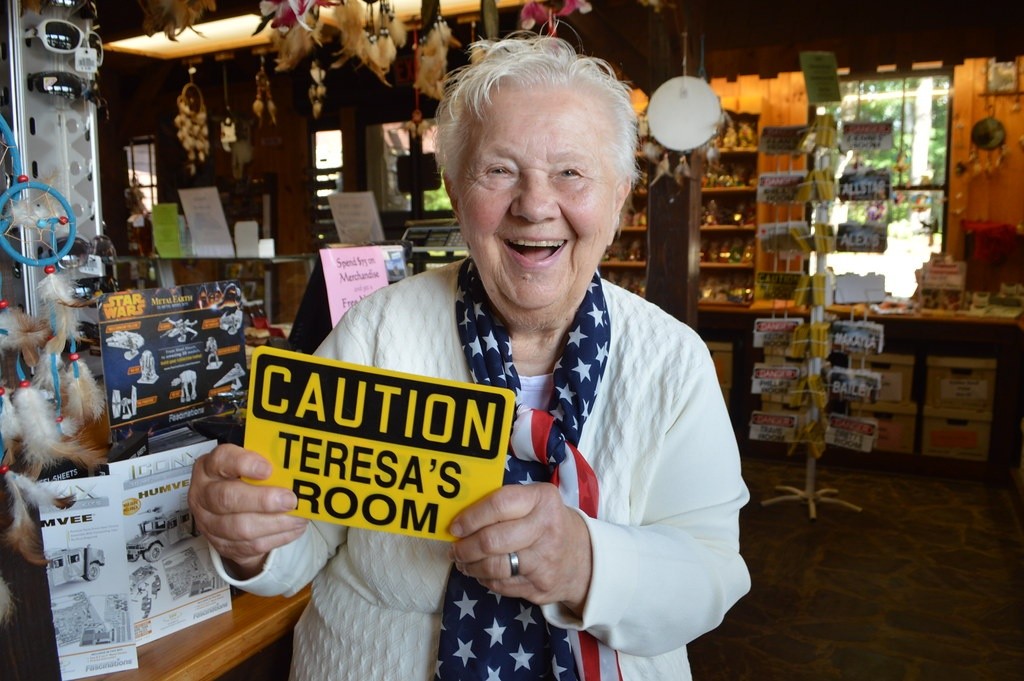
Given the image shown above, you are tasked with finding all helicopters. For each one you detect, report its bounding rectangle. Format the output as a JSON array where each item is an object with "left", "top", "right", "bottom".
[{"left": 159, "top": 317, "right": 198, "bottom": 343}]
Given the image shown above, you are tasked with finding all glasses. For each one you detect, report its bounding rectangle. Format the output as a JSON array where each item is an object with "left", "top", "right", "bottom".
[
  {"left": 63, "top": 320, "right": 102, "bottom": 354},
  {"left": 24, "top": 17, "right": 104, "bottom": 68},
  {"left": 69, "top": 274, "right": 121, "bottom": 302},
  {"left": 36, "top": 235, "right": 119, "bottom": 270},
  {"left": 39, "top": 0, "right": 99, "bottom": 20},
  {"left": 27, "top": 70, "right": 102, "bottom": 107}
]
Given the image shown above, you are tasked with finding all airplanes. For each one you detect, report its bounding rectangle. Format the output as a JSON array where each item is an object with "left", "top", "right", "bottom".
[{"left": 111, "top": 385, "right": 138, "bottom": 420}]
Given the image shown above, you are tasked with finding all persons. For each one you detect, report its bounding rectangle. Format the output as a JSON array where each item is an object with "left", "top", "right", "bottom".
[{"left": 189, "top": 31, "right": 751, "bottom": 680}]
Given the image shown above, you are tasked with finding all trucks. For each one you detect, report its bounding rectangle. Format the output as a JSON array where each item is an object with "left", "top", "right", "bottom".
[{"left": 45, "top": 545, "right": 107, "bottom": 586}]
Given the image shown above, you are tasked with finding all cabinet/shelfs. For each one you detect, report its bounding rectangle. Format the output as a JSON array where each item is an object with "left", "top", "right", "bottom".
[{"left": 593, "top": 107, "right": 764, "bottom": 314}]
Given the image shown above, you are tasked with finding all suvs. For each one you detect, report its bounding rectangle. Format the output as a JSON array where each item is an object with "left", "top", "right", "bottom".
[{"left": 125, "top": 509, "right": 201, "bottom": 562}]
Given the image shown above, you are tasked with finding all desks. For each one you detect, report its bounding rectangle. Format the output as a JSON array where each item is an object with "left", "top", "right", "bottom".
[
  {"left": 63, "top": 583, "right": 313, "bottom": 681},
  {"left": 116, "top": 252, "right": 319, "bottom": 326}
]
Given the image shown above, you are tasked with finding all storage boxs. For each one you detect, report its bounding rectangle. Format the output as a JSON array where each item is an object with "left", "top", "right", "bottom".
[{"left": 705, "top": 340, "right": 996, "bottom": 464}]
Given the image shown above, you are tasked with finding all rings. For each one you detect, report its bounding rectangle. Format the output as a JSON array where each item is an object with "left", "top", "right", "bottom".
[{"left": 508, "top": 551, "right": 521, "bottom": 578}]
